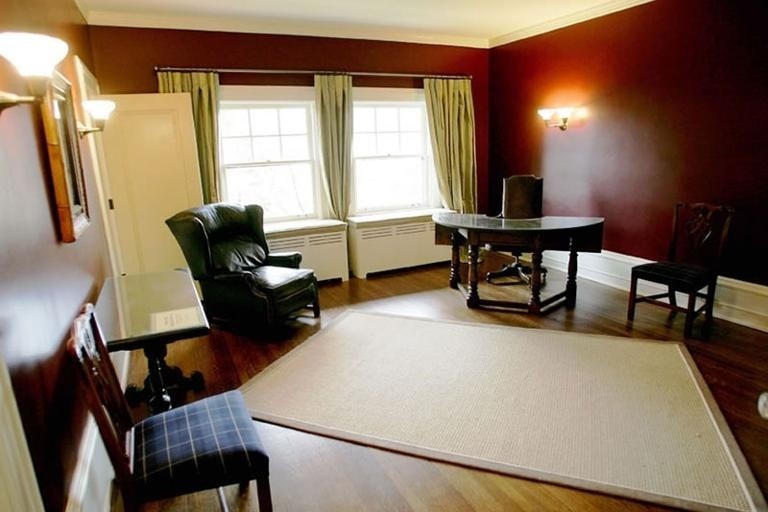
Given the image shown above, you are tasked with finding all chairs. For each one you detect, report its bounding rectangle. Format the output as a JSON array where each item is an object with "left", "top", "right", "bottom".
[
  {"left": 627, "top": 200, "right": 734, "bottom": 335},
  {"left": 65, "top": 303, "right": 272, "bottom": 510},
  {"left": 165, "top": 201, "right": 321, "bottom": 344},
  {"left": 484, "top": 173, "right": 549, "bottom": 286}
]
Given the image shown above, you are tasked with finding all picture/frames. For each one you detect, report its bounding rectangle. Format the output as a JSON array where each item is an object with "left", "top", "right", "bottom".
[{"left": 41, "top": 70, "right": 93, "bottom": 245}]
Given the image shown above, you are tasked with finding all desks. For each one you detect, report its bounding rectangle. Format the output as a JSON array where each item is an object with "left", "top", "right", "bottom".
[
  {"left": 91, "top": 267, "right": 212, "bottom": 415},
  {"left": 432, "top": 210, "right": 606, "bottom": 313}
]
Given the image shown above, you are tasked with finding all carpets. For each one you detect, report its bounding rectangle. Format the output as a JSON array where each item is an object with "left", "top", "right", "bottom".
[{"left": 237, "top": 308, "right": 767, "bottom": 511}]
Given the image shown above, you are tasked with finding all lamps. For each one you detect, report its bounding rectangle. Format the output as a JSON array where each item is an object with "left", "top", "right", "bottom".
[
  {"left": 537, "top": 107, "right": 573, "bottom": 132},
  {"left": 1, "top": 27, "right": 70, "bottom": 113},
  {"left": 76, "top": 100, "right": 116, "bottom": 140}
]
